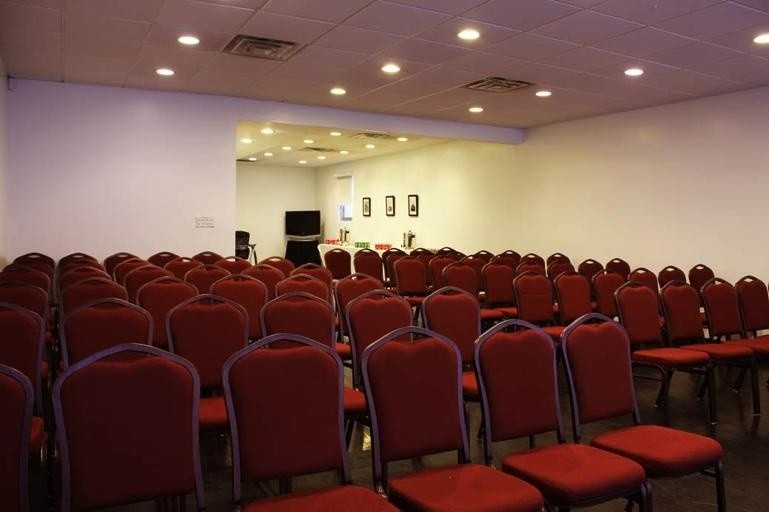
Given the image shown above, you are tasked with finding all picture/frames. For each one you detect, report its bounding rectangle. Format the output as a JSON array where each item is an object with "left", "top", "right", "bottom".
[
  {"left": 407, "top": 194, "right": 419, "bottom": 217},
  {"left": 385, "top": 195, "right": 396, "bottom": 217},
  {"left": 362, "top": 197, "right": 372, "bottom": 217}
]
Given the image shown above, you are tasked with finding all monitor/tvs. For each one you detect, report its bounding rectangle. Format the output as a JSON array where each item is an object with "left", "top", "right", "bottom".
[{"left": 283, "top": 208, "right": 324, "bottom": 242}]
